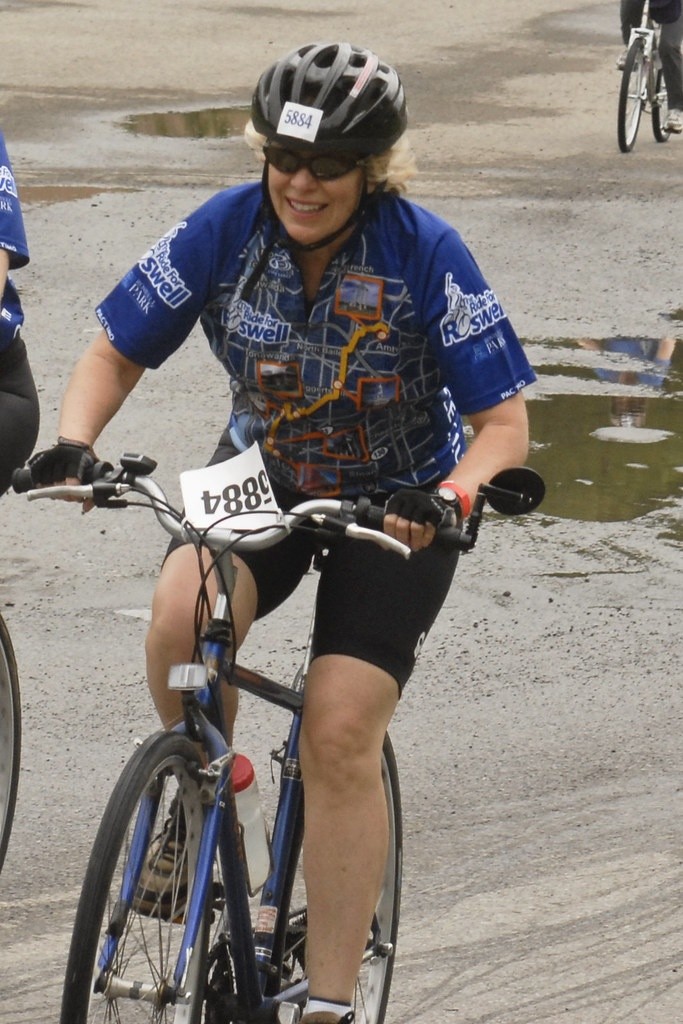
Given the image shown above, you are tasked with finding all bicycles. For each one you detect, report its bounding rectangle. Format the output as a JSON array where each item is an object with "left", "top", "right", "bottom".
[
  {"left": 13, "top": 452, "right": 552, "bottom": 1024},
  {"left": 615, "top": 0, "right": 673, "bottom": 153}
]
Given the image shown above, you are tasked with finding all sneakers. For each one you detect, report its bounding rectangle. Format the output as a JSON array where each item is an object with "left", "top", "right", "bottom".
[{"left": 131, "top": 791, "right": 214, "bottom": 924}]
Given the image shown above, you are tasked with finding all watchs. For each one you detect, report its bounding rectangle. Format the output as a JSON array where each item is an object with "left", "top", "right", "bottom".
[{"left": 433, "top": 488, "right": 462, "bottom": 531}]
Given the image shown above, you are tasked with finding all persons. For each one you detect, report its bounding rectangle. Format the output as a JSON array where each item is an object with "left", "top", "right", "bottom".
[
  {"left": 0, "top": 126, "right": 40, "bottom": 495},
  {"left": 616, "top": 0, "right": 683, "bottom": 131},
  {"left": 50, "top": 41, "right": 539, "bottom": 1024},
  {"left": 576, "top": 337, "right": 676, "bottom": 428}
]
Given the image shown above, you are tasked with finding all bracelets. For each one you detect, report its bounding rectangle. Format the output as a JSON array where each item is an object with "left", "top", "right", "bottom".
[{"left": 437, "top": 480, "right": 471, "bottom": 520}]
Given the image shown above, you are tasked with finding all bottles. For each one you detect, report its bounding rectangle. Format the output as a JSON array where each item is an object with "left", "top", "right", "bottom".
[
  {"left": 651, "top": 47, "right": 663, "bottom": 71},
  {"left": 216, "top": 751, "right": 271, "bottom": 893}
]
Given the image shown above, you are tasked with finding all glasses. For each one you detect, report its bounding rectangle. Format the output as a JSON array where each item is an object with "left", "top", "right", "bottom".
[{"left": 262, "top": 137, "right": 362, "bottom": 181}]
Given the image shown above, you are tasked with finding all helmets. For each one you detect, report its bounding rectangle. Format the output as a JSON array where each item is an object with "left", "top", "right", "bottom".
[{"left": 251, "top": 42, "right": 409, "bottom": 157}]
